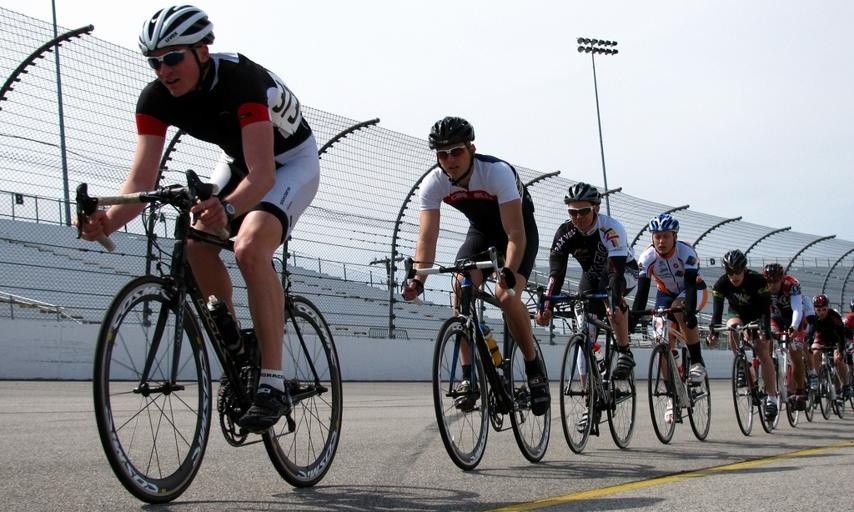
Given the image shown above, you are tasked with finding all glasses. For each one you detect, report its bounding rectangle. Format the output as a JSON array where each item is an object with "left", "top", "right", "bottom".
[
  {"left": 145, "top": 44, "right": 203, "bottom": 70},
  {"left": 816, "top": 308, "right": 828, "bottom": 312},
  {"left": 725, "top": 265, "right": 745, "bottom": 276},
  {"left": 435, "top": 144, "right": 468, "bottom": 162},
  {"left": 567, "top": 206, "right": 595, "bottom": 218}
]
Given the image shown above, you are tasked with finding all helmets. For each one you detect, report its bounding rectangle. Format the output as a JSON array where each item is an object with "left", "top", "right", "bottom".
[
  {"left": 722, "top": 249, "right": 747, "bottom": 270},
  {"left": 427, "top": 115, "right": 476, "bottom": 150},
  {"left": 136, "top": 3, "right": 215, "bottom": 58},
  {"left": 649, "top": 213, "right": 679, "bottom": 232},
  {"left": 563, "top": 182, "right": 601, "bottom": 204},
  {"left": 763, "top": 263, "right": 784, "bottom": 283},
  {"left": 812, "top": 294, "right": 829, "bottom": 307}
]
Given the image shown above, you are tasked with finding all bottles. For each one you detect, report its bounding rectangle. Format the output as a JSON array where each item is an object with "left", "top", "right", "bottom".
[
  {"left": 672, "top": 349, "right": 685, "bottom": 379},
  {"left": 201, "top": 294, "right": 246, "bottom": 354},
  {"left": 748, "top": 362, "right": 757, "bottom": 385},
  {"left": 480, "top": 322, "right": 503, "bottom": 368},
  {"left": 592, "top": 341, "right": 608, "bottom": 377}
]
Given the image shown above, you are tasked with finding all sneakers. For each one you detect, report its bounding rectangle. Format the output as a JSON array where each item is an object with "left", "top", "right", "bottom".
[{"left": 237, "top": 381, "right": 292, "bottom": 429}]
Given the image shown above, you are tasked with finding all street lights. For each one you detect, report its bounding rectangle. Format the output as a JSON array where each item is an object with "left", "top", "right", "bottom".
[{"left": 574, "top": 33, "right": 624, "bottom": 216}]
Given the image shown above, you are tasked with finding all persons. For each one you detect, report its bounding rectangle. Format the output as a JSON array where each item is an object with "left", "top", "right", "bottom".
[
  {"left": 704, "top": 250, "right": 779, "bottom": 418},
  {"left": 763, "top": 263, "right": 817, "bottom": 412},
  {"left": 78, "top": 3, "right": 322, "bottom": 431},
  {"left": 844, "top": 299, "right": 854, "bottom": 384},
  {"left": 802, "top": 294, "right": 850, "bottom": 398},
  {"left": 399, "top": 117, "right": 550, "bottom": 417},
  {"left": 629, "top": 214, "right": 710, "bottom": 425},
  {"left": 537, "top": 183, "right": 641, "bottom": 434}
]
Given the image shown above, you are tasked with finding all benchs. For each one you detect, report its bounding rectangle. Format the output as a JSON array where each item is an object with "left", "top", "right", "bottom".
[{"left": 696, "top": 263, "right": 854, "bottom": 323}]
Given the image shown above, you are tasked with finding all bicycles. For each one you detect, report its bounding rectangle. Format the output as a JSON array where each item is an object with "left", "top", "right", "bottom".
[
  {"left": 706, "top": 323, "right": 854, "bottom": 437},
  {"left": 635, "top": 305, "right": 712, "bottom": 445},
  {"left": 535, "top": 284, "right": 638, "bottom": 453},
  {"left": 403, "top": 245, "right": 553, "bottom": 470},
  {"left": 75, "top": 169, "right": 343, "bottom": 506}
]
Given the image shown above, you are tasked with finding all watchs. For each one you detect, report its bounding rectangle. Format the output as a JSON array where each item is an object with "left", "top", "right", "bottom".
[{"left": 222, "top": 200, "right": 236, "bottom": 222}]
